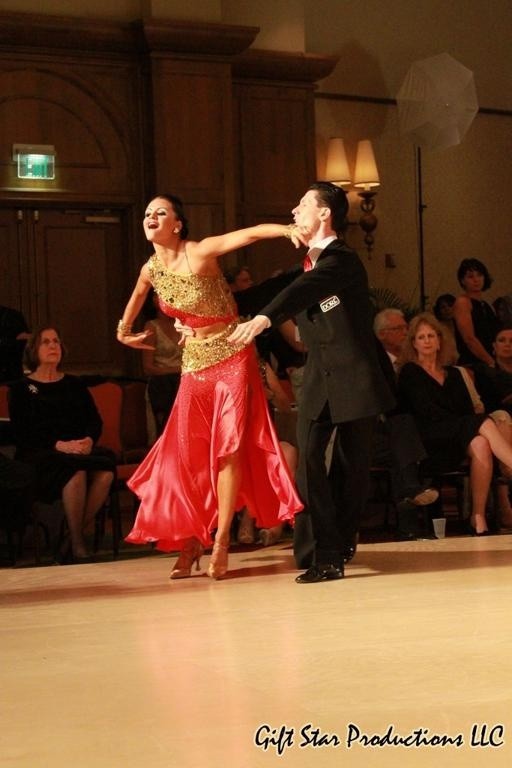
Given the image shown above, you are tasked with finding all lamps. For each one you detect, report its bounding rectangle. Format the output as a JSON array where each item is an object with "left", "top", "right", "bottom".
[{"left": 325, "top": 138, "right": 381, "bottom": 262}]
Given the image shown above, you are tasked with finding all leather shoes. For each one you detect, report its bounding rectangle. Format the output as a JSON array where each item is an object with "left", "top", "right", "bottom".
[{"left": 295, "top": 543, "right": 356, "bottom": 584}]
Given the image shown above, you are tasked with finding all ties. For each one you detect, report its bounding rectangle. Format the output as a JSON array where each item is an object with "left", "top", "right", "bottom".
[{"left": 302, "top": 252, "right": 312, "bottom": 271}]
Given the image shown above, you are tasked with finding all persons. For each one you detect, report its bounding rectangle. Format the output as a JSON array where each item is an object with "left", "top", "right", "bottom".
[
  {"left": 237, "top": 358, "right": 298, "bottom": 546},
  {"left": 376, "top": 255, "right": 512, "bottom": 540},
  {"left": 225, "top": 263, "right": 307, "bottom": 379},
  {"left": 134, "top": 290, "right": 184, "bottom": 447},
  {"left": 7, "top": 323, "right": 116, "bottom": 565},
  {"left": 115, "top": 193, "right": 312, "bottom": 581},
  {"left": 225, "top": 182, "right": 403, "bottom": 583},
  {"left": 1, "top": 305, "right": 28, "bottom": 383}
]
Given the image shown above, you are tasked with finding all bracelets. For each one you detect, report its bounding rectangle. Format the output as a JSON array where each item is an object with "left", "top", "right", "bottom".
[
  {"left": 283, "top": 222, "right": 298, "bottom": 239},
  {"left": 116, "top": 320, "right": 133, "bottom": 336}
]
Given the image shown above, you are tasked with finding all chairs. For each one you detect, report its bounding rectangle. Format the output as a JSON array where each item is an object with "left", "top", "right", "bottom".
[{"left": 87, "top": 382, "right": 157, "bottom": 560}]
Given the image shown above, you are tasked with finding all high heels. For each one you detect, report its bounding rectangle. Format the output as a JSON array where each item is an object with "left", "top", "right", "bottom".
[{"left": 168, "top": 542, "right": 229, "bottom": 578}]
